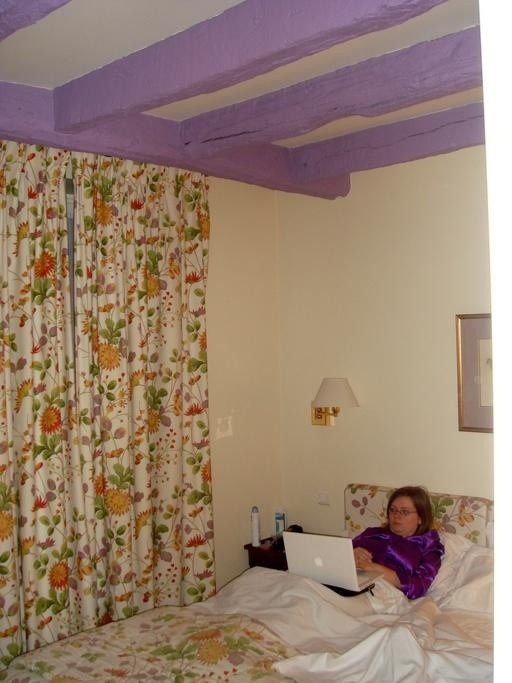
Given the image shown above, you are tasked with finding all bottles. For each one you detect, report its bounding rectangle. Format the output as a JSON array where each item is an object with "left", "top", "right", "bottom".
[
  {"left": 273, "top": 511, "right": 286, "bottom": 535},
  {"left": 249, "top": 506, "right": 261, "bottom": 548}
]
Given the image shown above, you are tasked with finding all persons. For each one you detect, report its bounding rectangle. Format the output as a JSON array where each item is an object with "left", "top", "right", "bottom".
[{"left": 347, "top": 484, "right": 446, "bottom": 602}]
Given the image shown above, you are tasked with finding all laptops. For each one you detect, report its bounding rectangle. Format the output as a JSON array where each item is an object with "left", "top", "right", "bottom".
[{"left": 282, "top": 531, "right": 385, "bottom": 592}]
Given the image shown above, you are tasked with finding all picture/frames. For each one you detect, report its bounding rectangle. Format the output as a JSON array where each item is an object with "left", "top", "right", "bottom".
[{"left": 456, "top": 314, "right": 492, "bottom": 433}]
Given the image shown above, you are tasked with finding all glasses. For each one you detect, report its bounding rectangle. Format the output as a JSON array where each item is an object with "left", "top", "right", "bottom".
[{"left": 389, "top": 506, "right": 418, "bottom": 516}]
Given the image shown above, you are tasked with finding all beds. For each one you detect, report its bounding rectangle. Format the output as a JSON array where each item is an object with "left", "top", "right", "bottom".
[{"left": 0, "top": 484, "right": 494, "bottom": 683}]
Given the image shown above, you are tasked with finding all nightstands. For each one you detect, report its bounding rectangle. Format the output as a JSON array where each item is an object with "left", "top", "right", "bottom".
[{"left": 244, "top": 535, "right": 289, "bottom": 571}]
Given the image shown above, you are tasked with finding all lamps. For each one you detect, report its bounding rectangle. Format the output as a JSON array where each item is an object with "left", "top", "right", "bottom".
[{"left": 309, "top": 378, "right": 359, "bottom": 426}]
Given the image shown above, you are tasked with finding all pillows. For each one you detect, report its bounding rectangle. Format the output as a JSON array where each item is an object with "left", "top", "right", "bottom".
[{"left": 425, "top": 530, "right": 493, "bottom": 614}]
